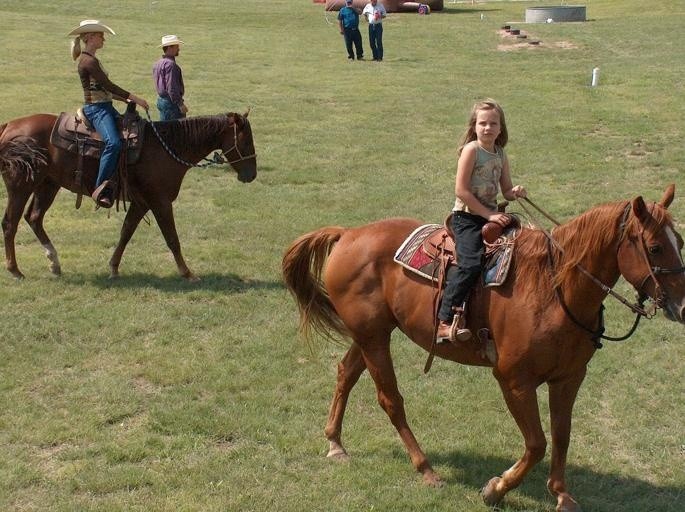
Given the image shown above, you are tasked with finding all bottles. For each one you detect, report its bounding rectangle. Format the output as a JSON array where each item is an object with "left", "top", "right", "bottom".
[
  {"left": 592, "top": 67, "right": 600, "bottom": 86},
  {"left": 481, "top": 13, "right": 484, "bottom": 20}
]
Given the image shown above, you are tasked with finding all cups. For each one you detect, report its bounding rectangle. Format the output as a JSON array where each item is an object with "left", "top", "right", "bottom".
[{"left": 375, "top": 13, "right": 379, "bottom": 21}]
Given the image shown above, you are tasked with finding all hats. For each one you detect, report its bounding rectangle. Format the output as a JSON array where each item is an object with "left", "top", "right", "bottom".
[
  {"left": 68, "top": 19, "right": 116, "bottom": 36},
  {"left": 158, "top": 35, "right": 185, "bottom": 48}
]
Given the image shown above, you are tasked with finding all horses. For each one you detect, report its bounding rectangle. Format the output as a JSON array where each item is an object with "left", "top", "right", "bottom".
[
  {"left": 281, "top": 183, "right": 685, "bottom": 512},
  {"left": 0, "top": 108, "right": 257, "bottom": 284}
]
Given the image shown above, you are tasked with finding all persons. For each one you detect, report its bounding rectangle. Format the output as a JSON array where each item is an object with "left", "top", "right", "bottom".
[
  {"left": 435, "top": 96, "right": 527, "bottom": 345},
  {"left": 337, "top": 0, "right": 365, "bottom": 61},
  {"left": 362, "top": 0, "right": 387, "bottom": 61},
  {"left": 152, "top": 35, "right": 188, "bottom": 121},
  {"left": 65, "top": 19, "right": 149, "bottom": 207}
]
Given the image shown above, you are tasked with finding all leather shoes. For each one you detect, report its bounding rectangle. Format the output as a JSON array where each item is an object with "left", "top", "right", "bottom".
[
  {"left": 100, "top": 194, "right": 113, "bottom": 208},
  {"left": 435, "top": 321, "right": 472, "bottom": 346}
]
